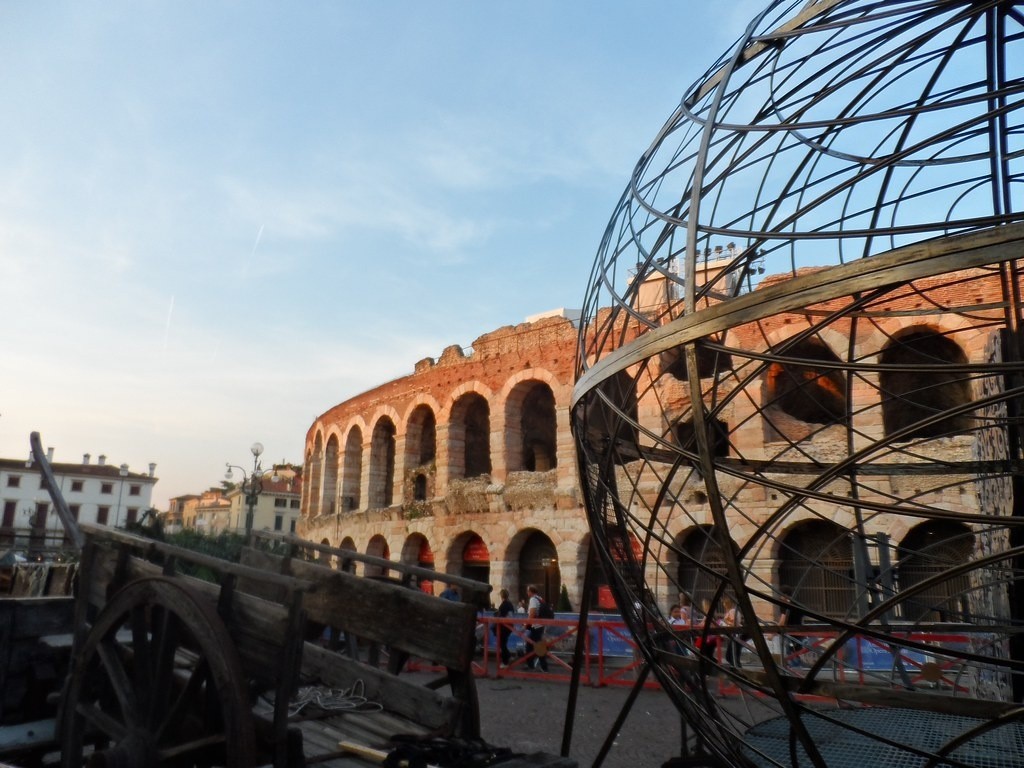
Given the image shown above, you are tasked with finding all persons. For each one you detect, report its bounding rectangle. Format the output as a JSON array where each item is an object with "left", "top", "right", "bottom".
[
  {"left": 766, "top": 586, "right": 803, "bottom": 670},
  {"left": 492, "top": 589, "right": 515, "bottom": 668},
  {"left": 517, "top": 585, "right": 550, "bottom": 674},
  {"left": 631, "top": 585, "right": 747, "bottom": 668},
  {"left": 438, "top": 583, "right": 462, "bottom": 603}
]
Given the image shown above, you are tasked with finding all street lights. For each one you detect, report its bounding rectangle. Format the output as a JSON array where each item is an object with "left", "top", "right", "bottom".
[{"left": 225, "top": 441, "right": 280, "bottom": 546}]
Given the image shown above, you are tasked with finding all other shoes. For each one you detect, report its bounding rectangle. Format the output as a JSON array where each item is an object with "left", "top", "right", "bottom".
[{"left": 500, "top": 663, "right": 508, "bottom": 669}]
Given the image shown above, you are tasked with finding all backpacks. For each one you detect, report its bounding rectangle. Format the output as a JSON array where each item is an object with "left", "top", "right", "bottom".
[{"left": 533, "top": 597, "right": 554, "bottom": 619}]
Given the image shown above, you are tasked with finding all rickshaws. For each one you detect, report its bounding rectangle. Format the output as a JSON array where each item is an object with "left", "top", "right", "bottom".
[{"left": 35, "top": 513, "right": 583, "bottom": 768}]
